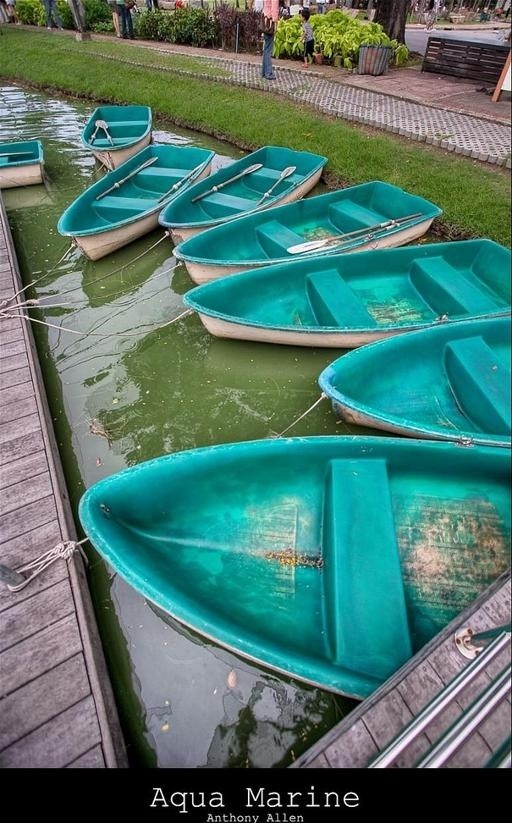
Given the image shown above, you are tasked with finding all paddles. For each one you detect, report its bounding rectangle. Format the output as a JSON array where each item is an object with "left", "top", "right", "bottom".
[
  {"left": 97, "top": 155, "right": 158, "bottom": 200},
  {"left": 257, "top": 166, "right": 297, "bottom": 205},
  {"left": 90, "top": 119, "right": 115, "bottom": 146},
  {"left": 191, "top": 163, "right": 263, "bottom": 204},
  {"left": 287, "top": 212, "right": 422, "bottom": 256}
]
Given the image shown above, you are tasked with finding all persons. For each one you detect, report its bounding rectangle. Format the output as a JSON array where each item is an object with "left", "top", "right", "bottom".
[
  {"left": 299, "top": 8, "right": 323, "bottom": 67},
  {"left": 254, "top": 0, "right": 280, "bottom": 80},
  {"left": 423, "top": 0, "right": 443, "bottom": 33},
  {"left": 115, "top": 0, "right": 139, "bottom": 40},
  {"left": 43, "top": 0, "right": 65, "bottom": 31},
  {"left": 0, "top": 0, "right": 23, "bottom": 25},
  {"left": 106, "top": 0, "right": 123, "bottom": 37}
]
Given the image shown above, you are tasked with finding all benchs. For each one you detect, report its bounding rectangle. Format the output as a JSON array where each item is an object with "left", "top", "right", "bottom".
[{"left": 421, "top": 36, "right": 512, "bottom": 84}]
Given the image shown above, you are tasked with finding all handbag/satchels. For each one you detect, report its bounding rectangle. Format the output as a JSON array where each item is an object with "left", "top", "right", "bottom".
[{"left": 258, "top": 12, "right": 276, "bottom": 36}]
